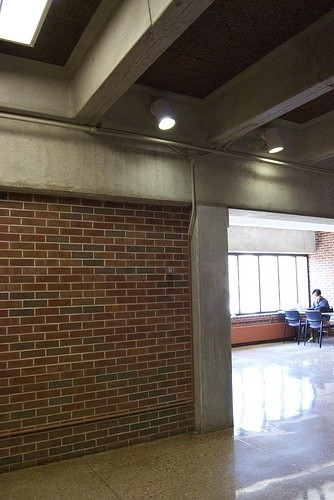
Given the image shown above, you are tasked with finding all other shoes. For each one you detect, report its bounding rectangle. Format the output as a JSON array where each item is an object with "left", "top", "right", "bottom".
[{"left": 308, "top": 336, "right": 316, "bottom": 343}]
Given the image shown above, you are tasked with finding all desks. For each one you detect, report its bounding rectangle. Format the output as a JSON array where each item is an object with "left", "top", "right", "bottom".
[{"left": 277, "top": 310, "right": 334, "bottom": 316}]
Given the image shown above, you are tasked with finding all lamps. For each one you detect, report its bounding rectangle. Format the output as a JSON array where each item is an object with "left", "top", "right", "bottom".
[
  {"left": 260, "top": 126, "right": 284, "bottom": 154},
  {"left": 150, "top": 96, "right": 176, "bottom": 130}
]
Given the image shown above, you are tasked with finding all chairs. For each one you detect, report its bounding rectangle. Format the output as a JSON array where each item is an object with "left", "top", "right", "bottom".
[
  {"left": 283, "top": 310, "right": 310, "bottom": 345},
  {"left": 304, "top": 310, "right": 334, "bottom": 348}
]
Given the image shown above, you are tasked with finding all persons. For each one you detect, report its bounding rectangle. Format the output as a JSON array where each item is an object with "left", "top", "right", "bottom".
[{"left": 302, "top": 289, "right": 331, "bottom": 343}]
